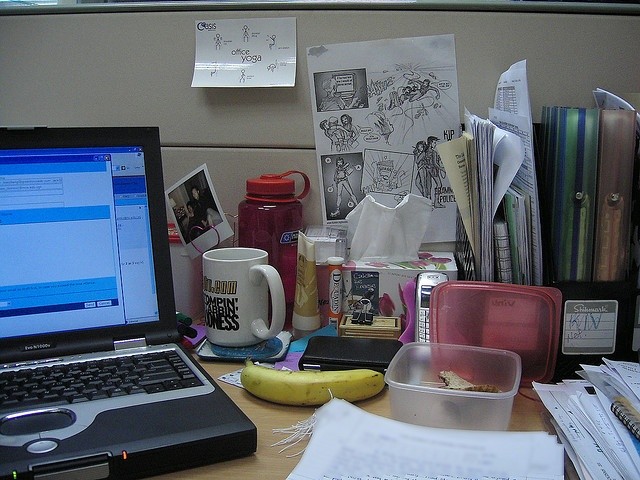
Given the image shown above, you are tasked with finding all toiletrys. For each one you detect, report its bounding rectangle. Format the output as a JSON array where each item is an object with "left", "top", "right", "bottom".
[{"left": 292, "top": 230, "right": 321, "bottom": 339}]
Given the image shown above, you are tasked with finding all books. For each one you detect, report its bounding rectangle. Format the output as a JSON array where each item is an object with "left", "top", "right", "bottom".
[
  {"left": 610, "top": 395, "right": 639, "bottom": 443},
  {"left": 495, "top": 219, "right": 513, "bottom": 282},
  {"left": 506, "top": 185, "right": 533, "bottom": 286}
]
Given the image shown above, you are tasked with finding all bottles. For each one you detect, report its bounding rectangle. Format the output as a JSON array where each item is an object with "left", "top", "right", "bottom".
[{"left": 238, "top": 169, "right": 310, "bottom": 335}]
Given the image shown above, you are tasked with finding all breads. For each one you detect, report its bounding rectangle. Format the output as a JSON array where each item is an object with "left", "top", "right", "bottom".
[{"left": 441, "top": 367, "right": 499, "bottom": 422}]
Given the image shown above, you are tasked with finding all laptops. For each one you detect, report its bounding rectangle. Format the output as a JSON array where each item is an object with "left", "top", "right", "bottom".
[{"left": 0, "top": 126, "right": 257, "bottom": 480}]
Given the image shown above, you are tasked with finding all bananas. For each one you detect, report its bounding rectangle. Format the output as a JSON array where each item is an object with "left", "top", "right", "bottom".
[{"left": 241, "top": 359, "right": 384, "bottom": 405}]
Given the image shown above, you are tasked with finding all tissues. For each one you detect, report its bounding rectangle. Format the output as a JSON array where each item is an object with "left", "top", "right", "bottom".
[{"left": 340, "top": 189, "right": 459, "bottom": 320}]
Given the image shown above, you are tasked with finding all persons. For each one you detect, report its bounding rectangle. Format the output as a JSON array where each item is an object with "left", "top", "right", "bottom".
[
  {"left": 319, "top": 114, "right": 359, "bottom": 154},
  {"left": 186, "top": 202, "right": 205, "bottom": 245},
  {"left": 191, "top": 185, "right": 222, "bottom": 227},
  {"left": 331, "top": 157, "right": 357, "bottom": 218},
  {"left": 405, "top": 78, "right": 440, "bottom": 102},
  {"left": 412, "top": 141, "right": 426, "bottom": 198},
  {"left": 426, "top": 135, "right": 445, "bottom": 209}
]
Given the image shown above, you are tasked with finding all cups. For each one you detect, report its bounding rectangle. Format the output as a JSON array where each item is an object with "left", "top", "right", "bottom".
[{"left": 202, "top": 247, "right": 286, "bottom": 348}]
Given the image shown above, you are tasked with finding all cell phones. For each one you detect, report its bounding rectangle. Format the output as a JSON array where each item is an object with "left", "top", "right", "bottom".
[{"left": 414, "top": 270, "right": 448, "bottom": 361}]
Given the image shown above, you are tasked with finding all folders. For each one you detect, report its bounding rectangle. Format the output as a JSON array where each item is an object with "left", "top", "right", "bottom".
[
  {"left": 593, "top": 111, "right": 636, "bottom": 282},
  {"left": 537, "top": 105, "right": 600, "bottom": 284}
]
